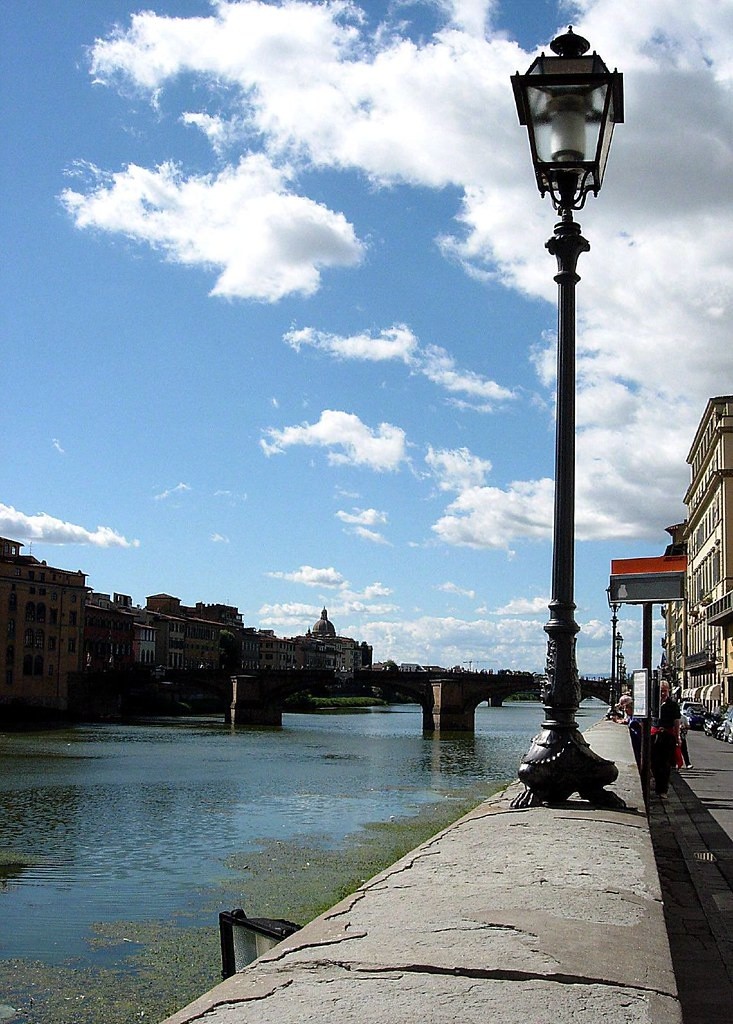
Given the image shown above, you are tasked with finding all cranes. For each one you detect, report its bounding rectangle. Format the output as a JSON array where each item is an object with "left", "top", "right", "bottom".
[{"left": 463, "top": 660, "right": 497, "bottom": 673}]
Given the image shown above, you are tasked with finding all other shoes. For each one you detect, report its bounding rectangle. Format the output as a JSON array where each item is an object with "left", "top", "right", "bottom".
[
  {"left": 659, "top": 793, "right": 667, "bottom": 799},
  {"left": 686, "top": 765, "right": 694, "bottom": 769}
]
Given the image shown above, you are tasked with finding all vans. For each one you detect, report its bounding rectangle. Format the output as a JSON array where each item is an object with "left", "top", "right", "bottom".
[{"left": 680, "top": 701, "right": 703, "bottom": 716}]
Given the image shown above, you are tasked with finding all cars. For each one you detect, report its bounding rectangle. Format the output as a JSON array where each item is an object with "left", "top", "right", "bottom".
[{"left": 680, "top": 706, "right": 704, "bottom": 729}]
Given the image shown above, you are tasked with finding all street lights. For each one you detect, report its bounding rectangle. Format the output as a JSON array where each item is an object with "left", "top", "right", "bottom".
[
  {"left": 510, "top": 26, "right": 624, "bottom": 809},
  {"left": 604, "top": 581, "right": 625, "bottom": 721}
]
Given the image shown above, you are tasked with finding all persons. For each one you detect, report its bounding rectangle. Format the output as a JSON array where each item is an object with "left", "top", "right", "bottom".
[
  {"left": 671, "top": 709, "right": 694, "bottom": 773},
  {"left": 650, "top": 680, "right": 680, "bottom": 799},
  {"left": 610, "top": 695, "right": 650, "bottom": 781}
]
